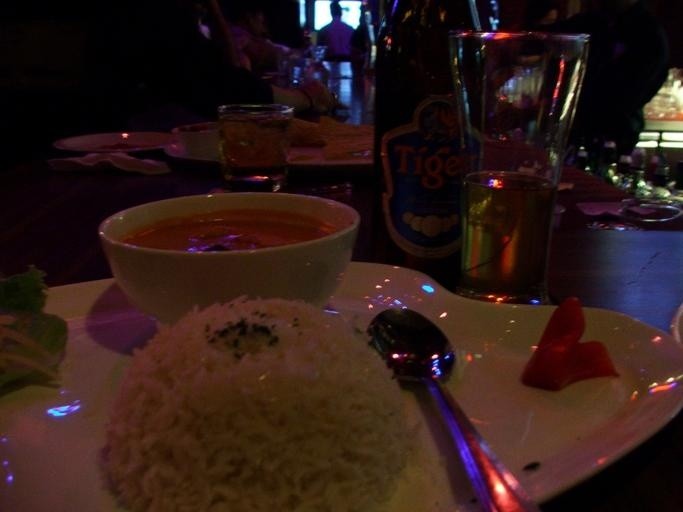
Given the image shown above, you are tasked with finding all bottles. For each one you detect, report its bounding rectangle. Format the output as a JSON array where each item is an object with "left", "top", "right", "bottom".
[
  {"left": 598, "top": 142, "right": 671, "bottom": 190},
  {"left": 362, "top": 2, "right": 497, "bottom": 273}
]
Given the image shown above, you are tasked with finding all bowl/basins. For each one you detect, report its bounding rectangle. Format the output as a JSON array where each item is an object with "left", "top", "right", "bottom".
[{"left": 95, "top": 191, "right": 361, "bottom": 327}]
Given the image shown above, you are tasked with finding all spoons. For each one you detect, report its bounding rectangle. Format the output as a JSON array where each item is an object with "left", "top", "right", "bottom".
[{"left": 367, "top": 302, "right": 538, "bottom": 511}]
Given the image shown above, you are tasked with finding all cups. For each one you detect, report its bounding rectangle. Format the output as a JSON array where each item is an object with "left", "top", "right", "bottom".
[
  {"left": 447, "top": 28, "right": 590, "bottom": 306},
  {"left": 218, "top": 100, "right": 293, "bottom": 191},
  {"left": 277, "top": 44, "right": 331, "bottom": 88}
]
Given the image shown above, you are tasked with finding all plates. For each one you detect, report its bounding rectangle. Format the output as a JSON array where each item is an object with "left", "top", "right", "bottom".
[
  {"left": 2, "top": 263, "right": 683, "bottom": 511},
  {"left": 54, "top": 122, "right": 374, "bottom": 168}
]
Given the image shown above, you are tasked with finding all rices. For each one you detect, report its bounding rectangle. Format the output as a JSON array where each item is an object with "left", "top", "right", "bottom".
[{"left": 105, "top": 295, "right": 407, "bottom": 512}]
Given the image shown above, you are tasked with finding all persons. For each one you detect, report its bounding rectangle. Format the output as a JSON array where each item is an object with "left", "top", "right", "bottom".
[{"left": 186, "top": 2, "right": 361, "bottom": 120}]
